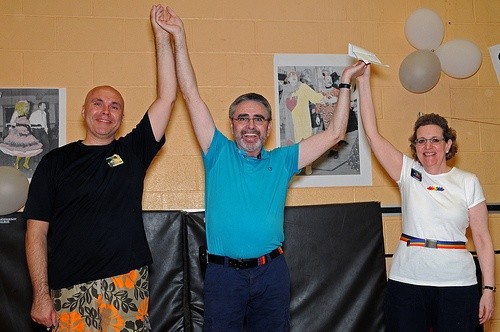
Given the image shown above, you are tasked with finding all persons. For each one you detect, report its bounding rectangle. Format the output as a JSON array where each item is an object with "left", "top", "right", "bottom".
[
  {"left": 0, "top": 100, "right": 43, "bottom": 169},
  {"left": 155, "top": 4, "right": 366, "bottom": 332},
  {"left": 355, "top": 63, "right": 495, "bottom": 332},
  {"left": 331, "top": 71, "right": 359, "bottom": 145},
  {"left": 279, "top": 72, "right": 339, "bottom": 175},
  {"left": 29, "top": 102, "right": 50, "bottom": 169},
  {"left": 316, "top": 76, "right": 340, "bottom": 158},
  {"left": 22, "top": 3, "right": 177, "bottom": 332}
]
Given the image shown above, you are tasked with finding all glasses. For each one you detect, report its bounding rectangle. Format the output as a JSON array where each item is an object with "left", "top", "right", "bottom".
[
  {"left": 232, "top": 117, "right": 270, "bottom": 123},
  {"left": 415, "top": 137, "right": 446, "bottom": 145}
]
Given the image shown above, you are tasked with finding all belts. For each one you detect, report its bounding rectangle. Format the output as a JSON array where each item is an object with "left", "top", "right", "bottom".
[{"left": 207, "top": 246, "right": 284, "bottom": 270}]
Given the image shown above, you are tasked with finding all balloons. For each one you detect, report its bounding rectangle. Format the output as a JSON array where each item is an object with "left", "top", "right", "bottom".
[
  {"left": 435, "top": 39, "right": 482, "bottom": 78},
  {"left": 404, "top": 8, "right": 444, "bottom": 51},
  {"left": 399, "top": 49, "right": 441, "bottom": 93}
]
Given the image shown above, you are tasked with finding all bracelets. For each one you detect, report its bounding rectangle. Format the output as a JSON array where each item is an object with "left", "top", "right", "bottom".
[{"left": 339, "top": 84, "right": 351, "bottom": 88}]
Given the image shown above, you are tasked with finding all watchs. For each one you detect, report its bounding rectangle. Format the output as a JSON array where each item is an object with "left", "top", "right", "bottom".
[{"left": 483, "top": 285, "right": 496, "bottom": 292}]
